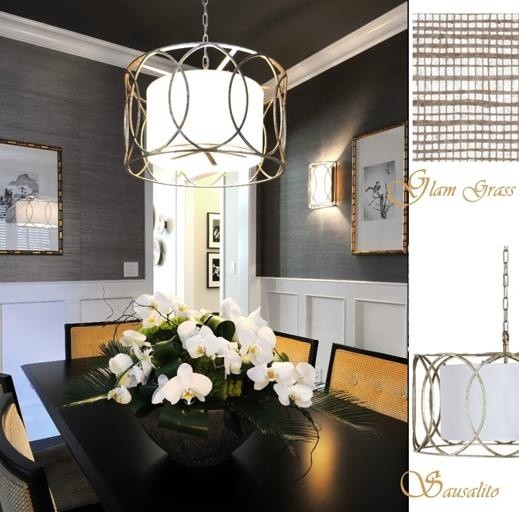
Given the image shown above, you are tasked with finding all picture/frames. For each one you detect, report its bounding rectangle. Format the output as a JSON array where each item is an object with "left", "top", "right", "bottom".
[
  {"left": 207, "top": 212, "right": 220, "bottom": 249},
  {"left": 0, "top": 139, "right": 63, "bottom": 255},
  {"left": 351, "top": 121, "right": 409, "bottom": 255},
  {"left": 207, "top": 252, "right": 220, "bottom": 288}
]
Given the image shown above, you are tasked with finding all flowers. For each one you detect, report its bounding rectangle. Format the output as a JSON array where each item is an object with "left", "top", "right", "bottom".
[{"left": 55, "top": 293, "right": 385, "bottom": 459}]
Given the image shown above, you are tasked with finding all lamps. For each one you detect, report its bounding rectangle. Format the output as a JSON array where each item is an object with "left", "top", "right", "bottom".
[
  {"left": 307, "top": 161, "right": 337, "bottom": 210},
  {"left": 413, "top": 247, "right": 519, "bottom": 457},
  {"left": 123, "top": 0, "right": 288, "bottom": 188},
  {"left": 16, "top": 150, "right": 58, "bottom": 230}
]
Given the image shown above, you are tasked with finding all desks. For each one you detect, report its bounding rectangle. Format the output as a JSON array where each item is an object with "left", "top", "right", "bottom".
[{"left": 20, "top": 360, "right": 408, "bottom": 512}]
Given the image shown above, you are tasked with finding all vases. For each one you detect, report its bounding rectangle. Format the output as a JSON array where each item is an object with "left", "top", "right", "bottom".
[{"left": 138, "top": 409, "right": 256, "bottom": 467}]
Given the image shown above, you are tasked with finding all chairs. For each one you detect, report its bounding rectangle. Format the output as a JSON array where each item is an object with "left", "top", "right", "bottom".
[
  {"left": 0, "top": 373, "right": 104, "bottom": 512},
  {"left": 273, "top": 331, "right": 408, "bottom": 424},
  {"left": 65, "top": 319, "right": 142, "bottom": 360}
]
